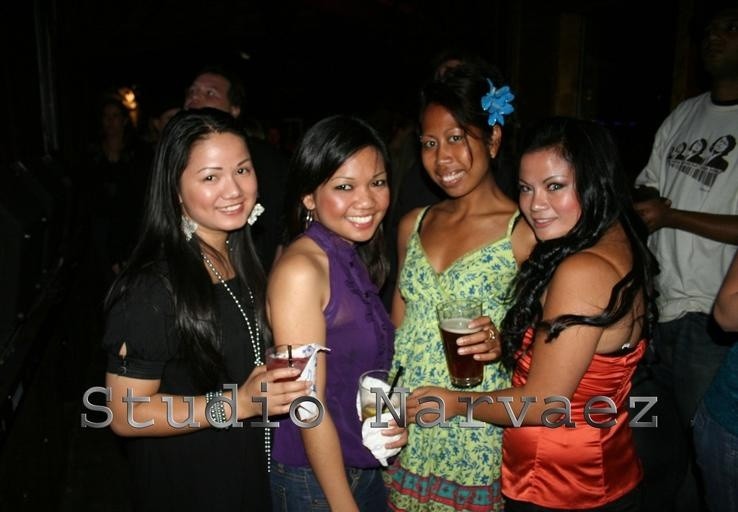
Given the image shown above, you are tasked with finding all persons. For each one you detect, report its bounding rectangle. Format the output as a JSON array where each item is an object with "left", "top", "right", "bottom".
[{"left": 68, "top": 0, "right": 738, "bottom": 512}]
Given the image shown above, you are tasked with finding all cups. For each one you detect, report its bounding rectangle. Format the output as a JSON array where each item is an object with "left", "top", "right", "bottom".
[
  {"left": 436, "top": 300, "right": 483, "bottom": 389},
  {"left": 265, "top": 345, "right": 316, "bottom": 382},
  {"left": 359, "top": 371, "right": 407, "bottom": 423}
]
